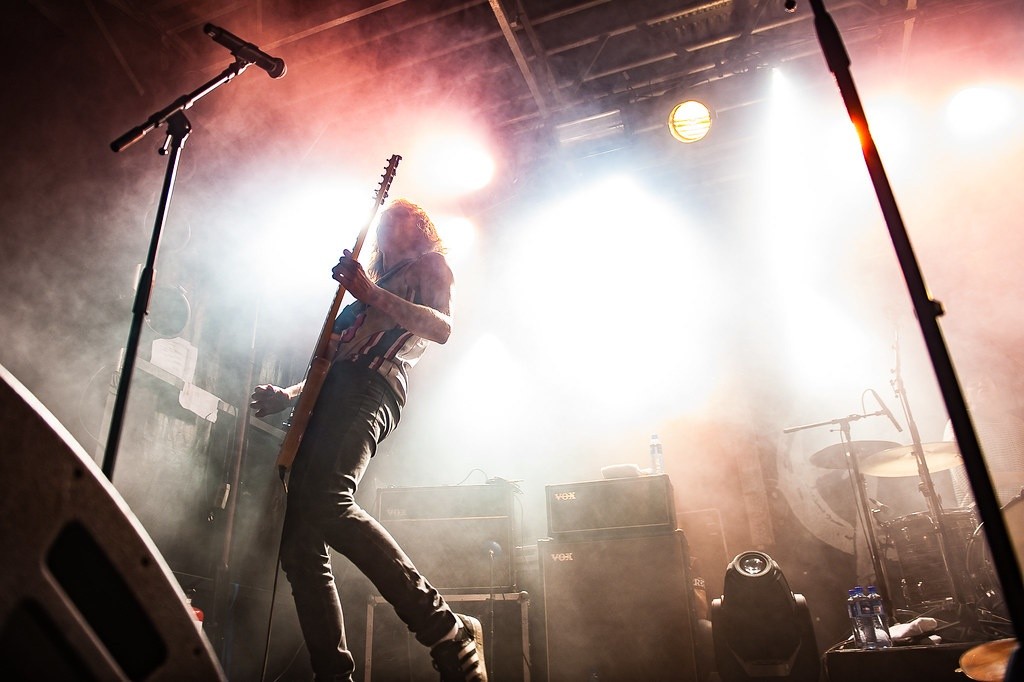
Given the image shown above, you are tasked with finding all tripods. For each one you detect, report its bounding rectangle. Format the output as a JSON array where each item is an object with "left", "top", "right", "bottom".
[{"left": 891, "top": 384, "right": 1013, "bottom": 646}]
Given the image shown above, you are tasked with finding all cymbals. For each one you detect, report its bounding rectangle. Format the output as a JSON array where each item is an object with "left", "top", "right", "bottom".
[
  {"left": 809, "top": 439, "right": 903, "bottom": 470},
  {"left": 856, "top": 440, "right": 965, "bottom": 478}
]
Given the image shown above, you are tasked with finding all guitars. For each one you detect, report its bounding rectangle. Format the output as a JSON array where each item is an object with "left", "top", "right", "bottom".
[{"left": 273, "top": 151, "right": 403, "bottom": 474}]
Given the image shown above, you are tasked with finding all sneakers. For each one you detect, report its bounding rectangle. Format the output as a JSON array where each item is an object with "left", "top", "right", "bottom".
[{"left": 431, "top": 615, "right": 487, "bottom": 682}]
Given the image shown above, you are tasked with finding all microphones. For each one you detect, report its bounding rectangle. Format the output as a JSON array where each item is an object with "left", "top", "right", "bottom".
[
  {"left": 869, "top": 497, "right": 890, "bottom": 515},
  {"left": 202, "top": 22, "right": 288, "bottom": 79}
]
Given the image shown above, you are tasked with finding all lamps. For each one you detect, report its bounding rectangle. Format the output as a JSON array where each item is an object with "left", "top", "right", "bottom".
[
  {"left": 667, "top": 94, "right": 720, "bottom": 145},
  {"left": 711, "top": 550, "right": 823, "bottom": 682}
]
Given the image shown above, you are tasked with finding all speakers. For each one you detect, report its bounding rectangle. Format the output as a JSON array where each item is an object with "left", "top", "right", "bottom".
[
  {"left": 374, "top": 483, "right": 519, "bottom": 592},
  {"left": 537, "top": 529, "right": 703, "bottom": 682}
]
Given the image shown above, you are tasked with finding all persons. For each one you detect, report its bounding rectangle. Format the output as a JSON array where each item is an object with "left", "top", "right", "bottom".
[{"left": 248, "top": 198, "right": 487, "bottom": 682}]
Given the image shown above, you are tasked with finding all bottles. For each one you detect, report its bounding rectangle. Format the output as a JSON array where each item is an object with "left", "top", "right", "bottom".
[
  {"left": 649, "top": 434, "right": 666, "bottom": 474},
  {"left": 847, "top": 586, "right": 893, "bottom": 649}
]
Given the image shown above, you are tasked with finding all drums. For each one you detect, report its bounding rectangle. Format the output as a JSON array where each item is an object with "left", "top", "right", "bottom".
[
  {"left": 880, "top": 506, "right": 986, "bottom": 612},
  {"left": 967, "top": 487, "right": 1024, "bottom": 636}
]
